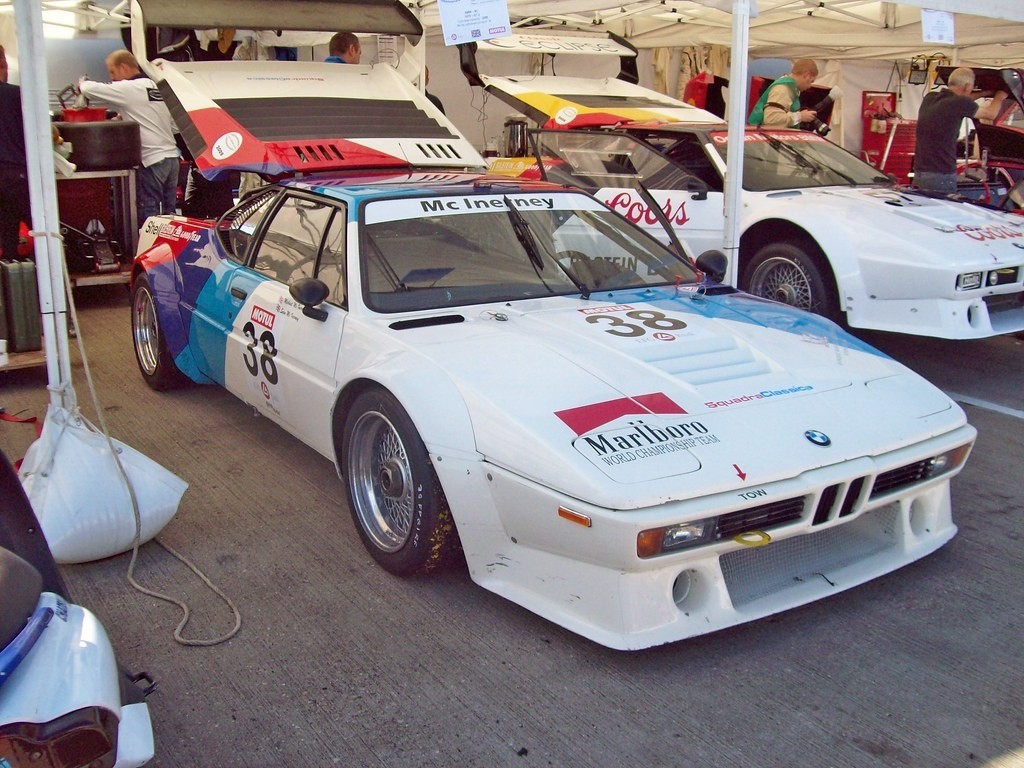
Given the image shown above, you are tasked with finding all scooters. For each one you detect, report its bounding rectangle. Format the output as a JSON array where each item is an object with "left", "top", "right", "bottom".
[{"left": 0, "top": 449, "right": 157, "bottom": 768}]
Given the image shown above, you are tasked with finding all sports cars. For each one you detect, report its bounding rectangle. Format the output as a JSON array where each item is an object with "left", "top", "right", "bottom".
[
  {"left": 456, "top": 30, "right": 1024, "bottom": 340},
  {"left": 129, "top": 0, "right": 978, "bottom": 651}
]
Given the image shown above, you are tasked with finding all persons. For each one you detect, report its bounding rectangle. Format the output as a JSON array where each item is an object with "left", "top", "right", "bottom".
[
  {"left": 748, "top": 58, "right": 819, "bottom": 129},
  {"left": 912, "top": 68, "right": 1009, "bottom": 195},
  {"left": 0, "top": 45, "right": 33, "bottom": 260},
  {"left": 182, "top": 165, "right": 236, "bottom": 220},
  {"left": 324, "top": 32, "right": 361, "bottom": 65},
  {"left": 77, "top": 48, "right": 180, "bottom": 226},
  {"left": 424, "top": 66, "right": 446, "bottom": 117}
]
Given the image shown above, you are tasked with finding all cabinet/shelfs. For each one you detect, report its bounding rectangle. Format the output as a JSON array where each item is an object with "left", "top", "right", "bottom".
[{"left": 56, "top": 169, "right": 140, "bottom": 286}]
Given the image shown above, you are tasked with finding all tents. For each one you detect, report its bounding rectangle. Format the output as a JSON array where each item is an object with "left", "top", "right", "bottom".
[{"left": 0, "top": 0, "right": 1023, "bottom": 415}]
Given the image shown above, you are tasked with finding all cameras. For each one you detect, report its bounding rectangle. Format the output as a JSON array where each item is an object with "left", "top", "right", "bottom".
[{"left": 802, "top": 85, "right": 844, "bottom": 136}]
